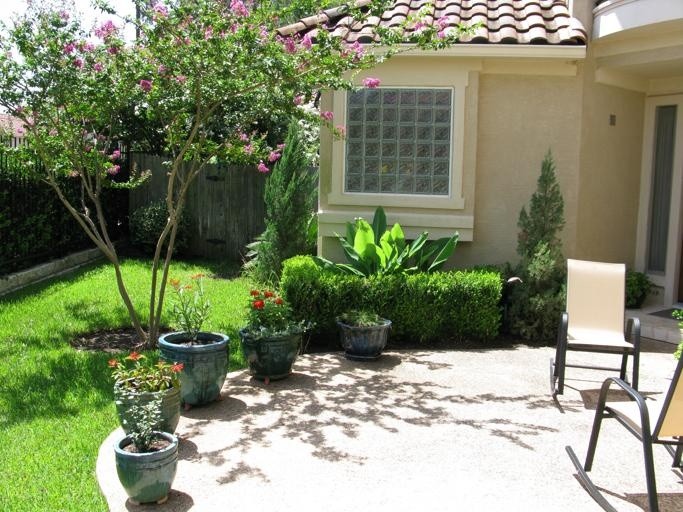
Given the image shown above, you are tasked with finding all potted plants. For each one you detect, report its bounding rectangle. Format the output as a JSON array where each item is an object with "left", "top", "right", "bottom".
[{"left": 334, "top": 311, "right": 393, "bottom": 362}]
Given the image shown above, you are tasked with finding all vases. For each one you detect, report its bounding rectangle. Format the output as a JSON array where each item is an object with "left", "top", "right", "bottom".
[
  {"left": 114, "top": 431, "right": 179, "bottom": 507},
  {"left": 158, "top": 330, "right": 231, "bottom": 413},
  {"left": 113, "top": 375, "right": 180, "bottom": 435},
  {"left": 239, "top": 329, "right": 302, "bottom": 382}
]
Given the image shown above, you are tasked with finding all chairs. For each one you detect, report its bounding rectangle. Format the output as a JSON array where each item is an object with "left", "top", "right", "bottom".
[{"left": 550, "top": 258, "right": 683, "bottom": 512}]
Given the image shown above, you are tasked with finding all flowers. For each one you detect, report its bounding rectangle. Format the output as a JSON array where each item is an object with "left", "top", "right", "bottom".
[
  {"left": 244, "top": 291, "right": 302, "bottom": 339},
  {"left": 106, "top": 352, "right": 184, "bottom": 396},
  {"left": 162, "top": 270, "right": 226, "bottom": 343},
  {"left": 119, "top": 398, "right": 165, "bottom": 451}
]
